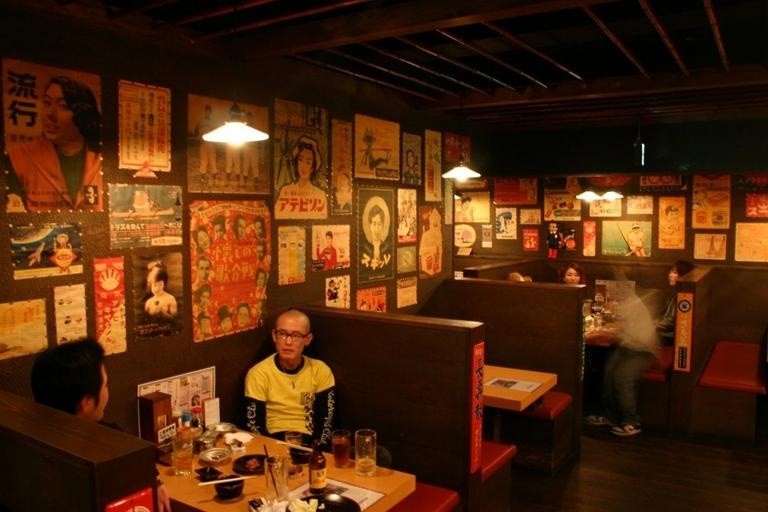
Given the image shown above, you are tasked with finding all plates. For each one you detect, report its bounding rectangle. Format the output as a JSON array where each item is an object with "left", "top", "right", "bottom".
[
  {"left": 297, "top": 494, "right": 362, "bottom": 512},
  {"left": 199, "top": 448, "right": 230, "bottom": 464},
  {"left": 233, "top": 453, "right": 267, "bottom": 474},
  {"left": 211, "top": 422, "right": 237, "bottom": 433}
]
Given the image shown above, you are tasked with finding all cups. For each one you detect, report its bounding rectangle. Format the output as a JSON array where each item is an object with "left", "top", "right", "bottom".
[
  {"left": 174, "top": 432, "right": 193, "bottom": 479},
  {"left": 582, "top": 294, "right": 615, "bottom": 333},
  {"left": 284, "top": 429, "right": 301, "bottom": 452},
  {"left": 355, "top": 428, "right": 377, "bottom": 476},
  {"left": 332, "top": 429, "right": 351, "bottom": 467},
  {"left": 264, "top": 454, "right": 288, "bottom": 503}
]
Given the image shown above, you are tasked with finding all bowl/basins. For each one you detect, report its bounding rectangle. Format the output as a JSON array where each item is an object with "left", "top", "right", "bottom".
[
  {"left": 195, "top": 466, "right": 223, "bottom": 481},
  {"left": 193, "top": 437, "right": 217, "bottom": 453},
  {"left": 289, "top": 446, "right": 310, "bottom": 463},
  {"left": 215, "top": 474, "right": 244, "bottom": 498}
]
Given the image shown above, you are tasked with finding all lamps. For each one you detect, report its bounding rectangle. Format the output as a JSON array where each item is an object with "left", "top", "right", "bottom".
[
  {"left": 442, "top": 44, "right": 481, "bottom": 183},
  {"left": 202, "top": 35, "right": 270, "bottom": 148}
]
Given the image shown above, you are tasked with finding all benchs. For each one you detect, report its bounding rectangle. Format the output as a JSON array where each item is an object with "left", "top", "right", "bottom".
[
  {"left": 444, "top": 276, "right": 587, "bottom": 476},
  {"left": 273, "top": 303, "right": 517, "bottom": 511},
  {"left": 636, "top": 264, "right": 766, "bottom": 439}
]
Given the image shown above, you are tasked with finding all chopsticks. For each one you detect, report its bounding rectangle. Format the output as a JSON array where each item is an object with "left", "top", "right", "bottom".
[{"left": 198, "top": 475, "right": 255, "bottom": 487}]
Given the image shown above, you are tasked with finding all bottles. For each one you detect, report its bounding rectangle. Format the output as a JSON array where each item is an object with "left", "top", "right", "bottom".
[
  {"left": 182, "top": 408, "right": 201, "bottom": 428},
  {"left": 310, "top": 443, "right": 327, "bottom": 494}
]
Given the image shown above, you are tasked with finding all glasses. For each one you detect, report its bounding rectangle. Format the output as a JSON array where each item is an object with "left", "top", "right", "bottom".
[{"left": 275, "top": 329, "right": 309, "bottom": 342}]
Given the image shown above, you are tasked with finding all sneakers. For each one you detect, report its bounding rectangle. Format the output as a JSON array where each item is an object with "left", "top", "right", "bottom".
[
  {"left": 611, "top": 423, "right": 642, "bottom": 436},
  {"left": 585, "top": 415, "right": 618, "bottom": 426}
]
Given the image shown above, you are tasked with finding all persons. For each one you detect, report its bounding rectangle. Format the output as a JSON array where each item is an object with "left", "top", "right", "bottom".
[
  {"left": 508, "top": 261, "right": 693, "bottom": 437},
  {"left": 192, "top": 216, "right": 268, "bottom": 338},
  {"left": 658, "top": 207, "right": 685, "bottom": 248},
  {"left": 545, "top": 222, "right": 562, "bottom": 259},
  {"left": 368, "top": 205, "right": 384, "bottom": 240},
  {"left": 244, "top": 310, "right": 336, "bottom": 449},
  {"left": 30, "top": 339, "right": 123, "bottom": 430},
  {"left": 327, "top": 280, "right": 337, "bottom": 300},
  {"left": 693, "top": 190, "right": 707, "bottom": 210},
  {"left": 6, "top": 75, "right": 103, "bottom": 207},
  {"left": 707, "top": 235, "right": 725, "bottom": 258},
  {"left": 334, "top": 173, "right": 351, "bottom": 212},
  {"left": 316, "top": 232, "right": 337, "bottom": 270},
  {"left": 270, "top": 136, "right": 328, "bottom": 218},
  {"left": 629, "top": 224, "right": 648, "bottom": 257},
  {"left": 144, "top": 271, "right": 177, "bottom": 323},
  {"left": 457, "top": 197, "right": 472, "bottom": 221},
  {"left": 401, "top": 148, "right": 419, "bottom": 184},
  {"left": 198, "top": 106, "right": 260, "bottom": 191},
  {"left": 526, "top": 236, "right": 536, "bottom": 249}
]
